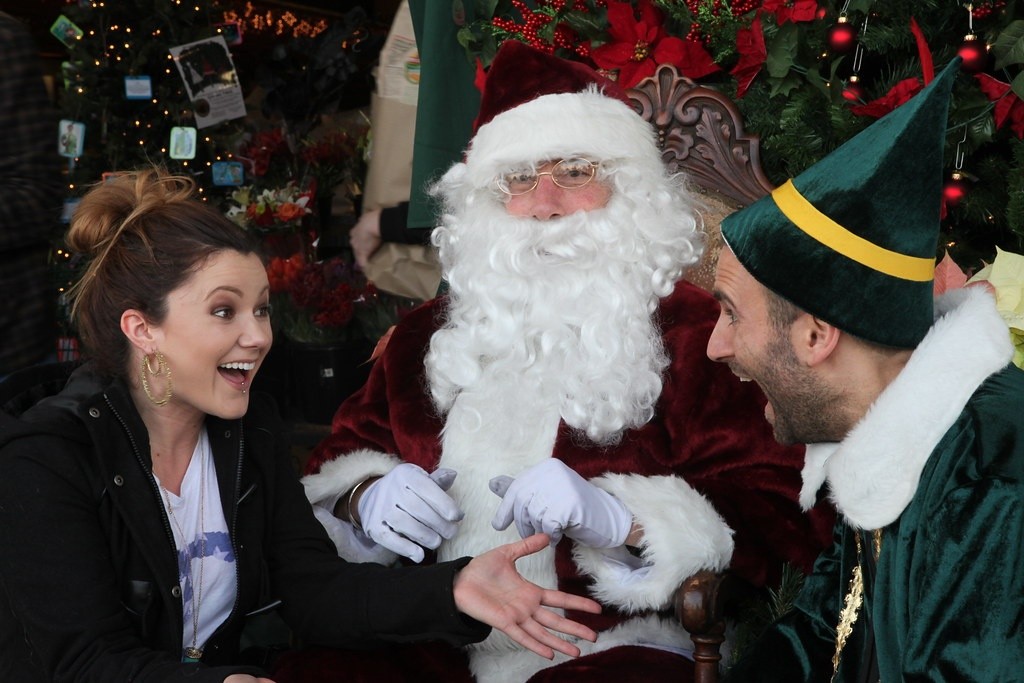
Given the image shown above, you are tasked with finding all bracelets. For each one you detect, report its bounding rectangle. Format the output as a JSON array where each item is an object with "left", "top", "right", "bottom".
[{"left": 346, "top": 474, "right": 370, "bottom": 530}]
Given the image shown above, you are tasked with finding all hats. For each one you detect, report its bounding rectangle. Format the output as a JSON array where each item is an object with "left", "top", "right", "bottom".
[
  {"left": 442, "top": 37, "right": 666, "bottom": 194},
  {"left": 719, "top": 52, "right": 967, "bottom": 347}
]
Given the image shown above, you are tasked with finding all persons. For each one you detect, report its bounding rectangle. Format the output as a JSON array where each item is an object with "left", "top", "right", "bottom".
[
  {"left": 702, "top": 239, "right": 1024, "bottom": 683},
  {"left": 1, "top": 163, "right": 600, "bottom": 683},
  {"left": 299, "top": 40, "right": 840, "bottom": 683}
]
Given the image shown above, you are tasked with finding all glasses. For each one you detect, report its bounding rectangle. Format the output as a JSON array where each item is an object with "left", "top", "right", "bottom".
[{"left": 494, "top": 160, "right": 604, "bottom": 197}]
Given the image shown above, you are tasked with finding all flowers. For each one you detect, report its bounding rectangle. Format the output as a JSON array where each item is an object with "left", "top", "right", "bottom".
[{"left": 221, "top": 117, "right": 381, "bottom": 349}]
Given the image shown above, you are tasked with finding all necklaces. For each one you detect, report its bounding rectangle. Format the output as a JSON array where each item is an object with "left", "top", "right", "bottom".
[{"left": 153, "top": 424, "right": 204, "bottom": 663}]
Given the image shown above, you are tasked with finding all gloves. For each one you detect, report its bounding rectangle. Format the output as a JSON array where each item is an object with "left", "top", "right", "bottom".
[
  {"left": 354, "top": 460, "right": 464, "bottom": 562},
  {"left": 488, "top": 456, "right": 636, "bottom": 550}
]
